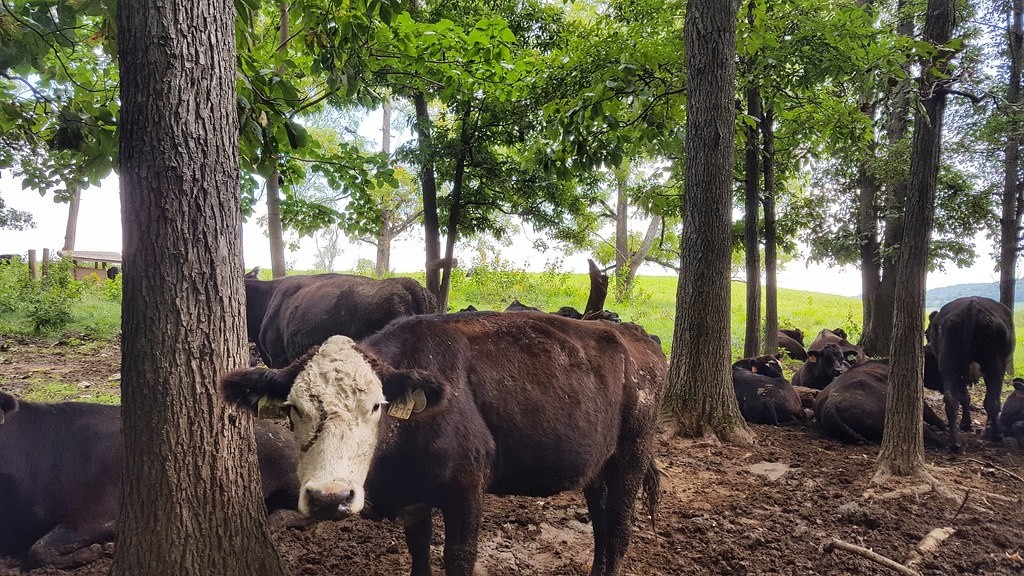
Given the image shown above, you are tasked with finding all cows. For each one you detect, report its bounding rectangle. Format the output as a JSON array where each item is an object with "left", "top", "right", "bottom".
[{"left": 0, "top": 266, "right": 1024, "bottom": 576}]
